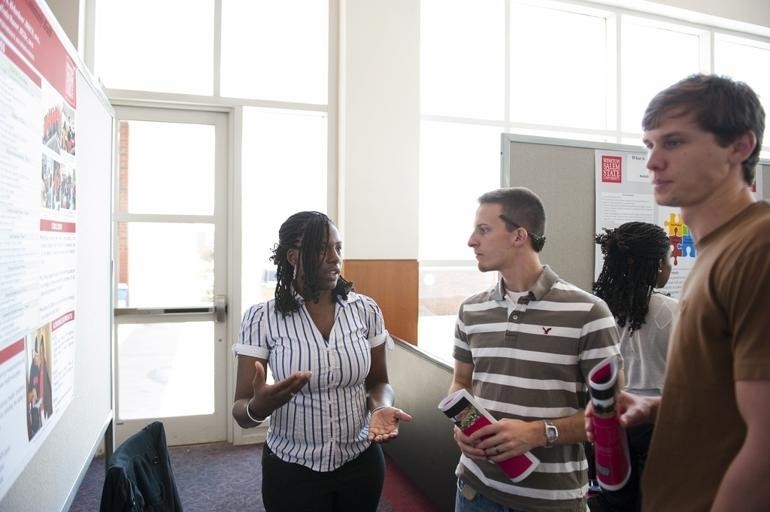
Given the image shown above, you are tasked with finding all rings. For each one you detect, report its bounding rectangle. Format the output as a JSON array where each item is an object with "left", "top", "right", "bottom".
[
  {"left": 290, "top": 392, "right": 297, "bottom": 398},
  {"left": 493, "top": 444, "right": 501, "bottom": 455}
]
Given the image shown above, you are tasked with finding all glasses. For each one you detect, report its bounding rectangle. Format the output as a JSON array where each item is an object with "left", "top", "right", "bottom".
[{"left": 499, "top": 214, "right": 547, "bottom": 251}]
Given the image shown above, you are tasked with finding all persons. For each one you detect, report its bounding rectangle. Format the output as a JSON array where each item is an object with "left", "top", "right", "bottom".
[
  {"left": 447, "top": 186, "right": 625, "bottom": 512},
  {"left": 36, "top": 102, "right": 79, "bottom": 209},
  {"left": 25, "top": 333, "right": 55, "bottom": 439},
  {"left": 585, "top": 73, "right": 770, "bottom": 512},
  {"left": 232, "top": 211, "right": 414, "bottom": 512},
  {"left": 591, "top": 220, "right": 683, "bottom": 511}
]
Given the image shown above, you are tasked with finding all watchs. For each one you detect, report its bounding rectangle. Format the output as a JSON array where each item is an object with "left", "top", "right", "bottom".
[{"left": 543, "top": 418, "right": 559, "bottom": 449}]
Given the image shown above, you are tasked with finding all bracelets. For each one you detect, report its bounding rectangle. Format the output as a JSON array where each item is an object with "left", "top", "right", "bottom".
[
  {"left": 371, "top": 406, "right": 391, "bottom": 418},
  {"left": 246, "top": 396, "right": 271, "bottom": 423}
]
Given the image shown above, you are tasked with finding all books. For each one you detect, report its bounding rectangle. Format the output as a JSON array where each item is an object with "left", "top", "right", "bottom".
[
  {"left": 584, "top": 356, "right": 634, "bottom": 492},
  {"left": 434, "top": 388, "right": 541, "bottom": 485}
]
